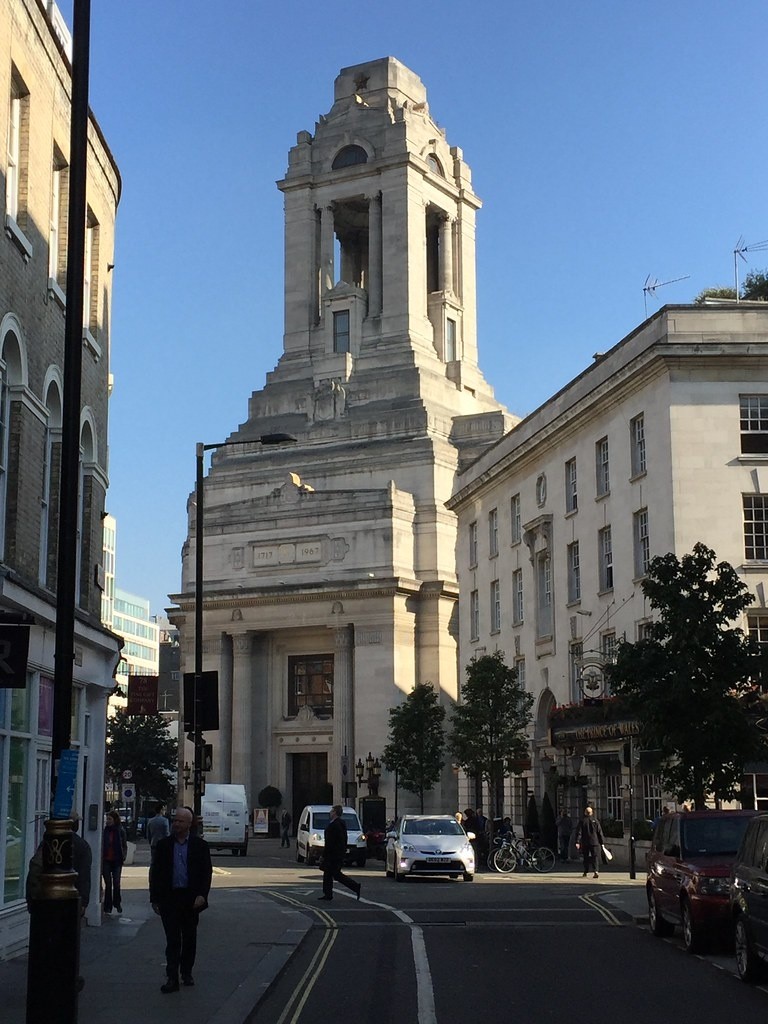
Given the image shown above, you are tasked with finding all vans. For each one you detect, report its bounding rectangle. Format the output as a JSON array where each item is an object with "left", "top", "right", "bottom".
[
  {"left": 297, "top": 805, "right": 367, "bottom": 869},
  {"left": 201, "top": 784, "right": 251, "bottom": 857},
  {"left": 113, "top": 807, "right": 135, "bottom": 828}
]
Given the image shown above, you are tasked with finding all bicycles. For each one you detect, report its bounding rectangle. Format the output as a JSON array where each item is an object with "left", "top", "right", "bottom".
[{"left": 487, "top": 830, "right": 556, "bottom": 873}]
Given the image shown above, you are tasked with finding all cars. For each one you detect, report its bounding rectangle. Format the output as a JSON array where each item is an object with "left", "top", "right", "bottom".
[{"left": 384, "top": 814, "right": 477, "bottom": 882}]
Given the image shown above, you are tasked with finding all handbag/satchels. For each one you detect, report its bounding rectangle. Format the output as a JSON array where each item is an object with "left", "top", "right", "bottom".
[{"left": 602, "top": 844, "right": 613, "bottom": 862}]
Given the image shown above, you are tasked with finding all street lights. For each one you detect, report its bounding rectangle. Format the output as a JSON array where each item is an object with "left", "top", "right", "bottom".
[
  {"left": 355, "top": 751, "right": 382, "bottom": 795},
  {"left": 192, "top": 432, "right": 300, "bottom": 834},
  {"left": 619, "top": 735, "right": 636, "bottom": 880}
]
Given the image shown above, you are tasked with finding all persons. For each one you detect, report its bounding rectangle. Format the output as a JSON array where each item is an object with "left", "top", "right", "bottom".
[
  {"left": 101, "top": 811, "right": 128, "bottom": 913},
  {"left": 456, "top": 808, "right": 516, "bottom": 873},
  {"left": 279, "top": 807, "right": 292, "bottom": 849},
  {"left": 150, "top": 808, "right": 212, "bottom": 993},
  {"left": 555, "top": 807, "right": 572, "bottom": 863},
  {"left": 317, "top": 804, "right": 362, "bottom": 901},
  {"left": 384, "top": 818, "right": 395, "bottom": 834},
  {"left": 575, "top": 806, "right": 606, "bottom": 878},
  {"left": 650, "top": 803, "right": 710, "bottom": 832},
  {"left": 184, "top": 806, "right": 202, "bottom": 838},
  {"left": 149, "top": 801, "right": 170, "bottom": 864}
]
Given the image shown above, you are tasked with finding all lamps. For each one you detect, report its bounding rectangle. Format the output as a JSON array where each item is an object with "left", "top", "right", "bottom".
[{"left": 576, "top": 609, "right": 592, "bottom": 616}]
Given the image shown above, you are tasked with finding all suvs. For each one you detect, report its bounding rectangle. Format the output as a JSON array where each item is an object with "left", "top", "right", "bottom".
[
  {"left": 646, "top": 810, "right": 764, "bottom": 947},
  {"left": 731, "top": 808, "right": 768, "bottom": 980}
]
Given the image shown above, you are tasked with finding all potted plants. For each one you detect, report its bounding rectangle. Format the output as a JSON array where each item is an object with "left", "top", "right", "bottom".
[{"left": 257, "top": 787, "right": 282, "bottom": 837}]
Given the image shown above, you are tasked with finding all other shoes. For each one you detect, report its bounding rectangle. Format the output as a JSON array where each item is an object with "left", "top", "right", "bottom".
[
  {"left": 592, "top": 870, "right": 598, "bottom": 878},
  {"left": 355, "top": 882, "right": 362, "bottom": 900},
  {"left": 317, "top": 895, "right": 333, "bottom": 902},
  {"left": 161, "top": 978, "right": 180, "bottom": 994},
  {"left": 114, "top": 901, "right": 122, "bottom": 913},
  {"left": 181, "top": 970, "right": 194, "bottom": 987}
]
